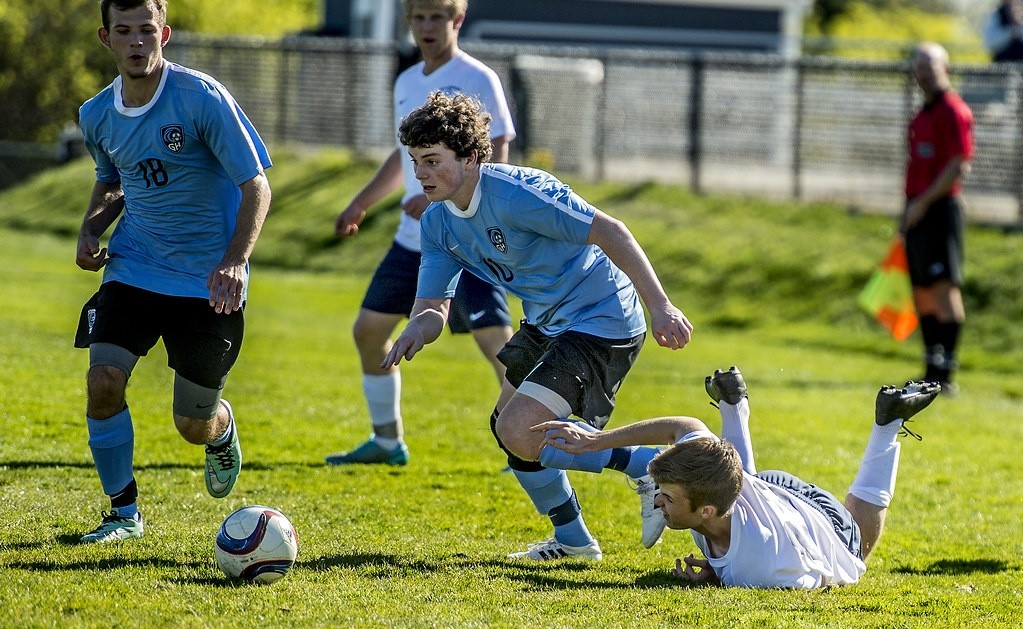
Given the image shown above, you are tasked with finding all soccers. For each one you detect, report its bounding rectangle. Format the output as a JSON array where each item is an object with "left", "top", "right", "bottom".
[{"left": 215, "top": 505, "right": 298, "bottom": 586}]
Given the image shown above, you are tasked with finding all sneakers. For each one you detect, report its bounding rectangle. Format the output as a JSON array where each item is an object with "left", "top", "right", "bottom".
[
  {"left": 504, "top": 535, "right": 602, "bottom": 562},
  {"left": 80, "top": 510, "right": 144, "bottom": 543},
  {"left": 875, "top": 380, "right": 941, "bottom": 441},
  {"left": 626, "top": 447, "right": 671, "bottom": 550},
  {"left": 326, "top": 438, "right": 408, "bottom": 466},
  {"left": 205, "top": 398, "right": 243, "bottom": 498},
  {"left": 705, "top": 365, "right": 748, "bottom": 409}
]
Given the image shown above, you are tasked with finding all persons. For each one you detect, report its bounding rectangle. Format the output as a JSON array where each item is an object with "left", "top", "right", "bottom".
[
  {"left": 528, "top": 360, "right": 945, "bottom": 599},
  {"left": 76, "top": 0, "right": 275, "bottom": 547},
  {"left": 890, "top": 37, "right": 973, "bottom": 391},
  {"left": 372, "top": 86, "right": 690, "bottom": 569},
  {"left": 331, "top": 2, "right": 546, "bottom": 472}
]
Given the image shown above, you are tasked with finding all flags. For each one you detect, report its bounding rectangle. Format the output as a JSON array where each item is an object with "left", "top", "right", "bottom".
[{"left": 856, "top": 235, "right": 921, "bottom": 343}]
